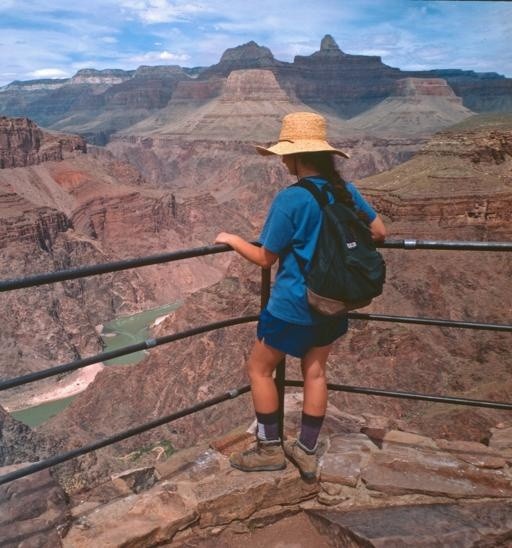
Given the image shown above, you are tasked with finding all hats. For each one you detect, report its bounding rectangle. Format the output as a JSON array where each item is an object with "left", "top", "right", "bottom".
[{"left": 256, "top": 111, "right": 350, "bottom": 159}]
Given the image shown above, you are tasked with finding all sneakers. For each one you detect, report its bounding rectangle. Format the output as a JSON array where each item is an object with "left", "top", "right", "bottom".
[{"left": 229, "top": 439, "right": 319, "bottom": 479}]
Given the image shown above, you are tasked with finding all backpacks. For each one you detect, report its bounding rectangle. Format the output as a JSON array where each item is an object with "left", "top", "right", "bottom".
[{"left": 288, "top": 180, "right": 386, "bottom": 317}]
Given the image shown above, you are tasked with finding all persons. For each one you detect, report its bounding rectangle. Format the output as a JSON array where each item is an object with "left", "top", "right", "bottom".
[{"left": 213, "top": 111, "right": 386, "bottom": 479}]
